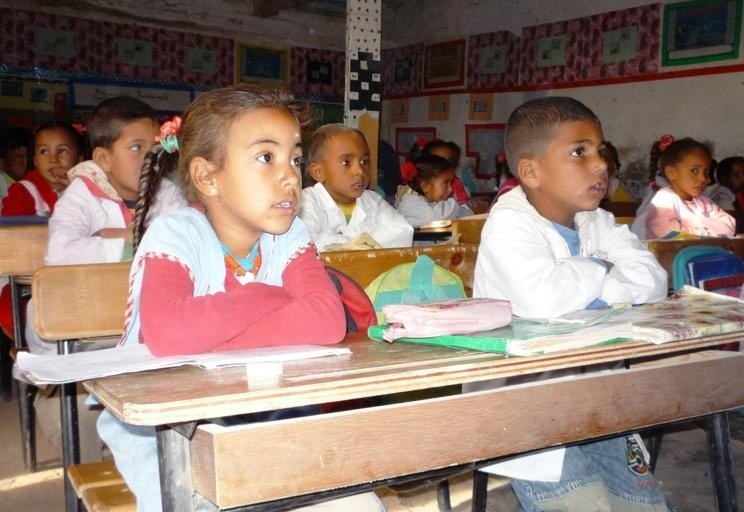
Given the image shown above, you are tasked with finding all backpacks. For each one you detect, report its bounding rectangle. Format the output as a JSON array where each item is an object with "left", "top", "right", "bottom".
[{"left": 363, "top": 256, "right": 467, "bottom": 325}]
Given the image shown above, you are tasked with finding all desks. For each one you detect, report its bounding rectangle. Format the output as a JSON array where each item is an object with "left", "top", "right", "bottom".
[{"left": 79, "top": 295, "right": 744, "bottom": 511}]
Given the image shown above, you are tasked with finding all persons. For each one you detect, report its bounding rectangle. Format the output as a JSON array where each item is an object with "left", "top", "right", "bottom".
[
  {"left": 394, "top": 139, "right": 468, "bottom": 202},
  {"left": 631, "top": 133, "right": 738, "bottom": 240},
  {"left": 296, "top": 123, "right": 415, "bottom": 252},
  {"left": 708, "top": 157, "right": 743, "bottom": 217},
  {"left": 25, "top": 94, "right": 188, "bottom": 464},
  {"left": 0, "top": 125, "right": 35, "bottom": 209},
  {"left": 0, "top": 119, "right": 91, "bottom": 344},
  {"left": 396, "top": 154, "right": 473, "bottom": 246},
  {"left": 90, "top": 80, "right": 384, "bottom": 508},
  {"left": 459, "top": 97, "right": 667, "bottom": 508}
]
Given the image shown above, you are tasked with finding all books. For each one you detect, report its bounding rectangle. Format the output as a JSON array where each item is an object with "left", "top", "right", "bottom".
[
  {"left": 686, "top": 254, "right": 743, "bottom": 352},
  {"left": 366, "top": 311, "right": 632, "bottom": 357},
  {"left": 12, "top": 343, "right": 354, "bottom": 392}
]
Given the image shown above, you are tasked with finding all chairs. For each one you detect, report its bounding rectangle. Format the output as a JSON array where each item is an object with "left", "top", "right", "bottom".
[
  {"left": 449, "top": 217, "right": 488, "bottom": 245},
  {"left": 1, "top": 223, "right": 53, "bottom": 474},
  {"left": 32, "top": 262, "right": 133, "bottom": 512}
]
